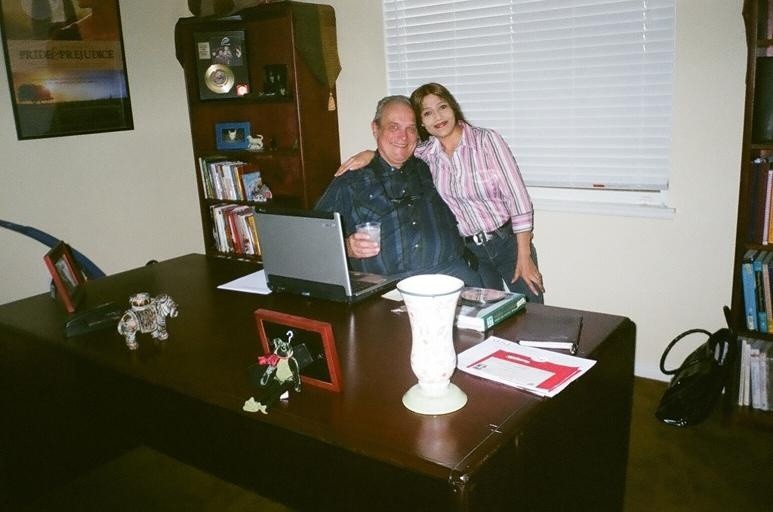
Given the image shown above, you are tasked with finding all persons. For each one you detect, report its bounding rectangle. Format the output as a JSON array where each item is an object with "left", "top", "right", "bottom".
[
  {"left": 310, "top": 95, "right": 485, "bottom": 290},
  {"left": 211, "top": 46, "right": 241, "bottom": 66},
  {"left": 333, "top": 81, "right": 546, "bottom": 305}
]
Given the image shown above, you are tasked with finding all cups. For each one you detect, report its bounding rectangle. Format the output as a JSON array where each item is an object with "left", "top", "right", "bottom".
[{"left": 354, "top": 222, "right": 381, "bottom": 252}]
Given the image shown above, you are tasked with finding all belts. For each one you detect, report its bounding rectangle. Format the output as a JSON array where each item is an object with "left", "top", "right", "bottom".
[{"left": 461, "top": 217, "right": 511, "bottom": 246}]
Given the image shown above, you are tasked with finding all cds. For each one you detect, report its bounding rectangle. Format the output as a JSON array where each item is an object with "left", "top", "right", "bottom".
[{"left": 461, "top": 288, "right": 505, "bottom": 303}]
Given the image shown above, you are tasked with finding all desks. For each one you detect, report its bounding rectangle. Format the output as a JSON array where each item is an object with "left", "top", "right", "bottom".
[{"left": 0, "top": 255, "right": 638, "bottom": 512}]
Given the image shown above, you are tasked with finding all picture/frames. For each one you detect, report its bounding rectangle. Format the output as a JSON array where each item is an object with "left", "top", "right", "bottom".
[{"left": 0, "top": 2, "right": 135, "bottom": 142}]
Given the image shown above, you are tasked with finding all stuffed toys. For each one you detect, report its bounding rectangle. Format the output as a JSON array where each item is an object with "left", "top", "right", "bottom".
[{"left": 239, "top": 330, "right": 304, "bottom": 414}]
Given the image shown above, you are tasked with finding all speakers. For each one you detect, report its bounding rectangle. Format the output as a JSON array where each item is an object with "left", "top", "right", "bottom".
[{"left": 193, "top": 31, "right": 251, "bottom": 100}]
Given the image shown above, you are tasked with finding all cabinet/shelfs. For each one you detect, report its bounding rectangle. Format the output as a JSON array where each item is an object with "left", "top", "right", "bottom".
[
  {"left": 175, "top": 0, "right": 341, "bottom": 260},
  {"left": 724, "top": 1, "right": 771, "bottom": 431}
]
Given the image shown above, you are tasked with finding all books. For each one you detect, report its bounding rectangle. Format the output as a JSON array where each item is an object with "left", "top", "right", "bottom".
[
  {"left": 195, "top": 154, "right": 272, "bottom": 255},
  {"left": 454, "top": 286, "right": 527, "bottom": 334},
  {"left": 761, "top": 169, "right": 773, "bottom": 246},
  {"left": 762, "top": 251, "right": 773, "bottom": 335},
  {"left": 767, "top": 255, "right": 773, "bottom": 315},
  {"left": 752, "top": 251, "right": 768, "bottom": 335},
  {"left": 767, "top": 180, "right": 773, "bottom": 243},
  {"left": 514, "top": 304, "right": 583, "bottom": 352},
  {"left": 736, "top": 248, "right": 760, "bottom": 334},
  {"left": 467, "top": 350, "right": 581, "bottom": 394},
  {"left": 737, "top": 337, "right": 772, "bottom": 414}
]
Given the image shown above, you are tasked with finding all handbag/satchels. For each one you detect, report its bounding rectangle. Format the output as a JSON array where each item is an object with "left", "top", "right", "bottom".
[{"left": 655, "top": 305, "right": 734, "bottom": 428}]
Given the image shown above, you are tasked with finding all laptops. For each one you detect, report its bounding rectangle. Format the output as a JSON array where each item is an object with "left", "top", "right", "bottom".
[{"left": 252, "top": 205, "right": 401, "bottom": 305}]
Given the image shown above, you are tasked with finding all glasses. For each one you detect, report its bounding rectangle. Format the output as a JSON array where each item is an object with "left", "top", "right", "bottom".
[{"left": 379, "top": 169, "right": 423, "bottom": 203}]
